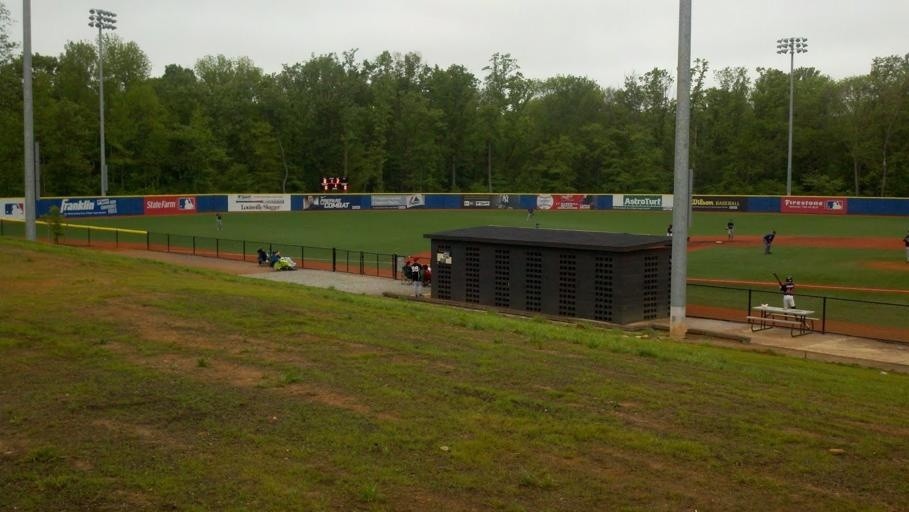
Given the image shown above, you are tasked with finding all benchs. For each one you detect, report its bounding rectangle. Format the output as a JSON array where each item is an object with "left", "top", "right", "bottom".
[
  {"left": 765, "top": 313, "right": 820, "bottom": 336},
  {"left": 746, "top": 316, "right": 802, "bottom": 337}
]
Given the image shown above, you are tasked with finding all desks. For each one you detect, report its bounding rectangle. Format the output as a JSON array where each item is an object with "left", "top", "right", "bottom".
[{"left": 752, "top": 306, "right": 815, "bottom": 335}]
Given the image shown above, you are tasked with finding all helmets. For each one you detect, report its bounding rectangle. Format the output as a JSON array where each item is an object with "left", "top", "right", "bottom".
[{"left": 786, "top": 277, "right": 792, "bottom": 282}]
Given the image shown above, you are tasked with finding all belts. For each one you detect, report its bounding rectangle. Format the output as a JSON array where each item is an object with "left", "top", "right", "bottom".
[{"left": 784, "top": 294, "right": 791, "bottom": 295}]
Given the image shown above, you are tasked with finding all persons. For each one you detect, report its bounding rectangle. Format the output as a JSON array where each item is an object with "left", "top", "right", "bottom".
[
  {"left": 727, "top": 218, "right": 736, "bottom": 241},
  {"left": 763, "top": 230, "right": 777, "bottom": 255},
  {"left": 403, "top": 256, "right": 431, "bottom": 284},
  {"left": 666, "top": 224, "right": 672, "bottom": 236},
  {"left": 215, "top": 212, "right": 224, "bottom": 232},
  {"left": 304, "top": 195, "right": 319, "bottom": 209},
  {"left": 903, "top": 230, "right": 909, "bottom": 262},
  {"left": 779, "top": 276, "right": 799, "bottom": 321},
  {"left": 257, "top": 248, "right": 296, "bottom": 270}
]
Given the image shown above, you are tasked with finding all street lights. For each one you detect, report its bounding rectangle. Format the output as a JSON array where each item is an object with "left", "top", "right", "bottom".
[
  {"left": 776, "top": 38, "right": 808, "bottom": 196},
  {"left": 89, "top": 8, "right": 116, "bottom": 196}
]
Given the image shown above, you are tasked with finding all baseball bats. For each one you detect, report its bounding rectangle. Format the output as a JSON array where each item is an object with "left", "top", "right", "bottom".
[{"left": 773, "top": 272, "right": 783, "bottom": 287}]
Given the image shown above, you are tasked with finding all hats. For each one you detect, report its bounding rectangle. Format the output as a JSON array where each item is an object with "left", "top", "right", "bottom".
[{"left": 413, "top": 258, "right": 418, "bottom": 261}]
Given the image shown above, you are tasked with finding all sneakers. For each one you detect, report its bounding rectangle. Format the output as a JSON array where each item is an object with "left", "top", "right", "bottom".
[{"left": 415, "top": 293, "right": 424, "bottom": 298}]
